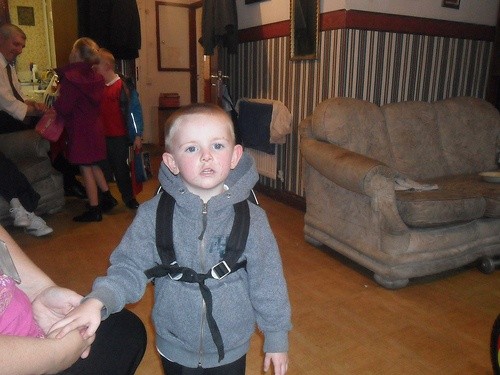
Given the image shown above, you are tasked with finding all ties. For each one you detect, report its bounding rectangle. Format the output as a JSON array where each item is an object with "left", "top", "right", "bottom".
[{"left": 6, "top": 65, "right": 24, "bottom": 103}]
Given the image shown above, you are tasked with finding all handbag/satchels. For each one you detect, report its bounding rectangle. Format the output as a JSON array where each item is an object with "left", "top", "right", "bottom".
[{"left": 35, "top": 110, "right": 64, "bottom": 142}]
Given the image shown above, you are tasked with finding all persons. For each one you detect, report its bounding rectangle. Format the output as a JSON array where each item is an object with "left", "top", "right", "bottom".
[
  {"left": 0, "top": 24, "right": 87, "bottom": 201},
  {"left": 0, "top": 223, "right": 148, "bottom": 375},
  {"left": 0, "top": 153, "right": 53, "bottom": 237},
  {"left": 48, "top": 102, "right": 293, "bottom": 375},
  {"left": 50, "top": 38, "right": 142, "bottom": 222}
]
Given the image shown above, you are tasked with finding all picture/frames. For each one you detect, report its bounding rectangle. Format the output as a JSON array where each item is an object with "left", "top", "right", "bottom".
[
  {"left": 441, "top": 0, "right": 460, "bottom": 10},
  {"left": 289, "top": 0, "right": 321, "bottom": 61}
]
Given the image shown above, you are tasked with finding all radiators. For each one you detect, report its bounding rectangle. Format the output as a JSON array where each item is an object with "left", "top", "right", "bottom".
[{"left": 237, "top": 98, "right": 284, "bottom": 182}]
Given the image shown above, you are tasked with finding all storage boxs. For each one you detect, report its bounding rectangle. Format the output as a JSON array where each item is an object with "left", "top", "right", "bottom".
[{"left": 159, "top": 93, "right": 180, "bottom": 107}]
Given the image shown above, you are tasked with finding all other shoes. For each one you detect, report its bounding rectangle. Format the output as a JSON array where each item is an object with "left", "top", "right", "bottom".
[
  {"left": 73, "top": 209, "right": 102, "bottom": 221},
  {"left": 122, "top": 194, "right": 139, "bottom": 209},
  {"left": 101, "top": 199, "right": 117, "bottom": 212}
]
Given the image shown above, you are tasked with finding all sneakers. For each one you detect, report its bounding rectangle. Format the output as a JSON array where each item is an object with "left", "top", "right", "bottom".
[
  {"left": 24, "top": 216, "right": 54, "bottom": 237},
  {"left": 9, "top": 206, "right": 30, "bottom": 226}
]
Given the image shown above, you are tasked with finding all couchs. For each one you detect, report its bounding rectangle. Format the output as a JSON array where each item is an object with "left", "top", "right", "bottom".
[{"left": 299, "top": 97, "right": 499, "bottom": 289}]
